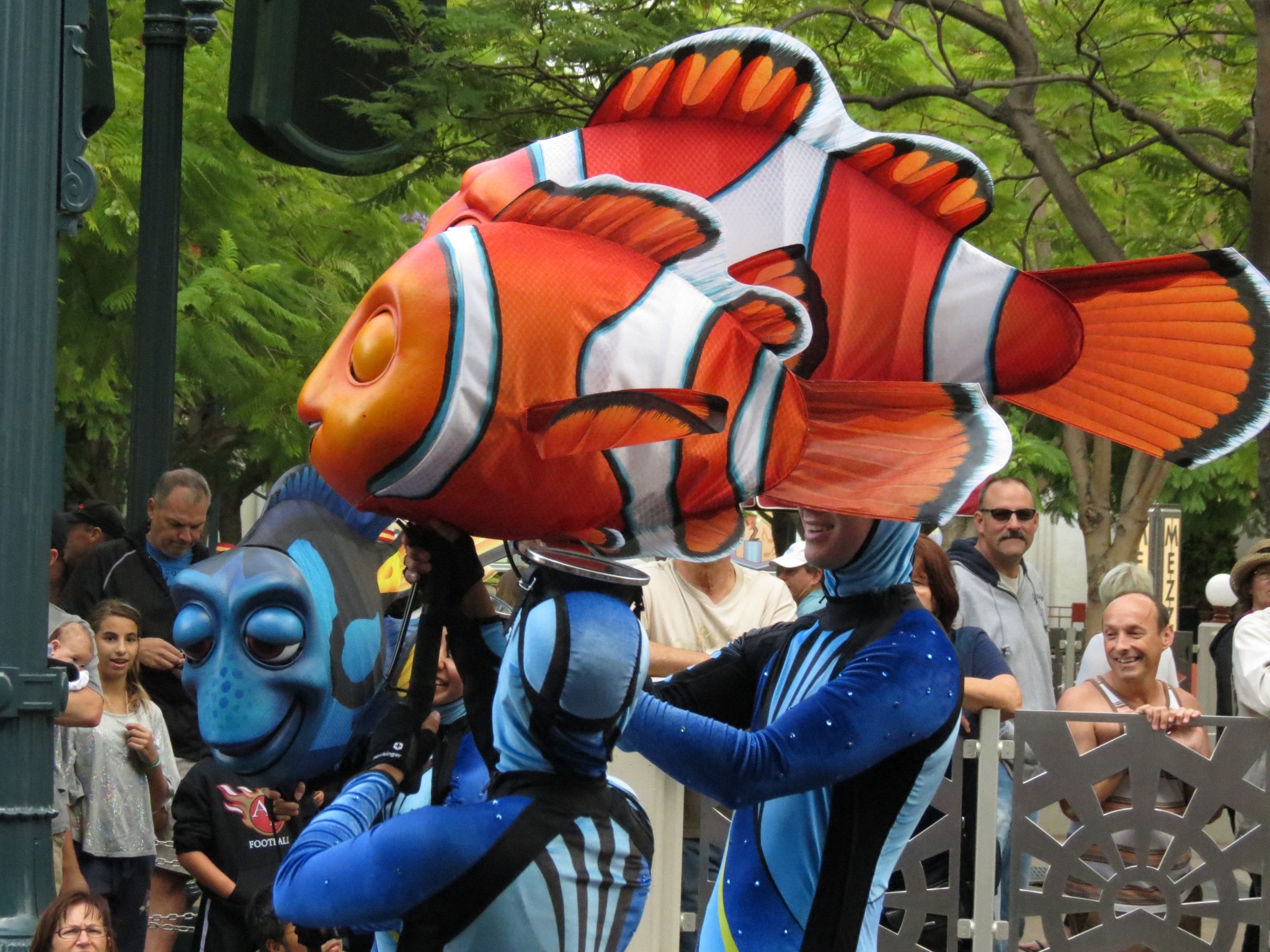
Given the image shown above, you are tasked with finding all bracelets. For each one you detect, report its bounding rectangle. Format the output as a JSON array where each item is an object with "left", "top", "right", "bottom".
[{"left": 143, "top": 753, "right": 160, "bottom": 768}]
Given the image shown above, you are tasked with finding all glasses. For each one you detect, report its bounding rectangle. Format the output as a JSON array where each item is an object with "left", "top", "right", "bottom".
[
  {"left": 980, "top": 508, "right": 1037, "bottom": 521},
  {"left": 54, "top": 925, "right": 108, "bottom": 939}
]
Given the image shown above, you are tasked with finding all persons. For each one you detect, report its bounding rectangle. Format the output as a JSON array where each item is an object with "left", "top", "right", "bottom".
[
  {"left": 51, "top": 498, "right": 133, "bottom": 620},
  {"left": 382, "top": 631, "right": 490, "bottom": 824},
  {"left": 29, "top": 890, "right": 117, "bottom": 952},
  {"left": 1057, "top": 589, "right": 1223, "bottom": 940},
  {"left": 171, "top": 756, "right": 375, "bottom": 952},
  {"left": 948, "top": 476, "right": 1057, "bottom": 952},
  {"left": 632, "top": 556, "right": 799, "bottom": 952},
  {"left": 261, "top": 518, "right": 655, "bottom": 952},
  {"left": 769, "top": 541, "right": 827, "bottom": 616},
  {"left": 47, "top": 622, "right": 95, "bottom": 690},
  {"left": 1076, "top": 563, "right": 1181, "bottom": 687},
  {"left": 62, "top": 598, "right": 184, "bottom": 952},
  {"left": 1209, "top": 538, "right": 1270, "bottom": 834},
  {"left": 55, "top": 467, "right": 212, "bottom": 952},
  {"left": 50, "top": 549, "right": 106, "bottom": 896},
  {"left": 1231, "top": 605, "right": 1270, "bottom": 952},
  {"left": 618, "top": 506, "right": 964, "bottom": 952},
  {"left": 884, "top": 536, "right": 1021, "bottom": 952}
]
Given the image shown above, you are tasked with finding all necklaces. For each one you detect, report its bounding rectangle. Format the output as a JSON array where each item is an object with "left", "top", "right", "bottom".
[{"left": 104, "top": 685, "right": 129, "bottom": 723}]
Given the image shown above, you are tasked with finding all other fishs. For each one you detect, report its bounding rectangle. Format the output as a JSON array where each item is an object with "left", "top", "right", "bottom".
[
  {"left": 171, "top": 461, "right": 420, "bottom": 786},
  {"left": 297, "top": 26, "right": 1269, "bottom": 567}
]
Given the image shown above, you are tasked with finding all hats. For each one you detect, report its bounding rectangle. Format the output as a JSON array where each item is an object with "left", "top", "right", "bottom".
[
  {"left": 1230, "top": 538, "right": 1270, "bottom": 597},
  {"left": 62, "top": 501, "right": 126, "bottom": 536},
  {"left": 768, "top": 541, "right": 808, "bottom": 568}
]
